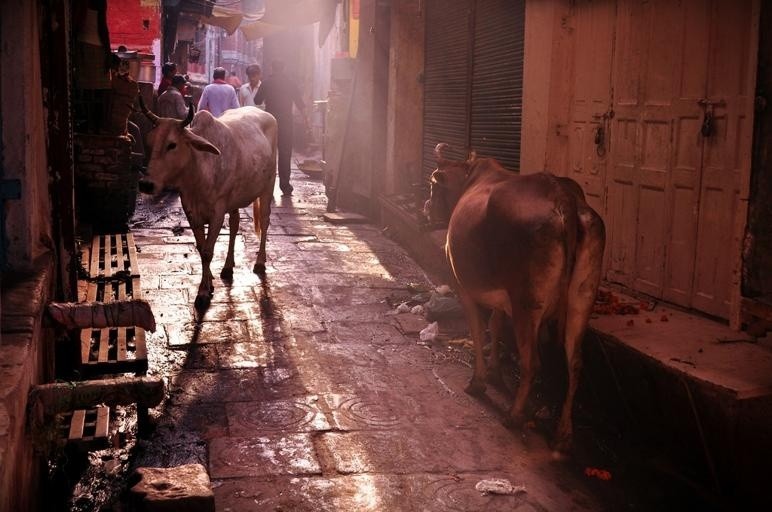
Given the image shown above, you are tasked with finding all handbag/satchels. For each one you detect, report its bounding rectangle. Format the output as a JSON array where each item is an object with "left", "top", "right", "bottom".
[{"left": 293, "top": 129, "right": 319, "bottom": 158}]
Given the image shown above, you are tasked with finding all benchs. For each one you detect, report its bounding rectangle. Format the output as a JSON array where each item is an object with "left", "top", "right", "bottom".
[{"left": 63, "top": 227, "right": 154, "bottom": 446}]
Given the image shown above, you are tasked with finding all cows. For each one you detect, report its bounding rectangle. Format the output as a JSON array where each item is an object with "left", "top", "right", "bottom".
[
  {"left": 134, "top": 92, "right": 283, "bottom": 314},
  {"left": 421, "top": 141, "right": 609, "bottom": 452}
]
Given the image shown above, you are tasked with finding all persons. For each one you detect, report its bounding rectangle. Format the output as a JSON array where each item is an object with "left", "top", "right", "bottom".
[
  {"left": 253, "top": 60, "right": 311, "bottom": 195},
  {"left": 155, "top": 60, "right": 265, "bottom": 123}
]
